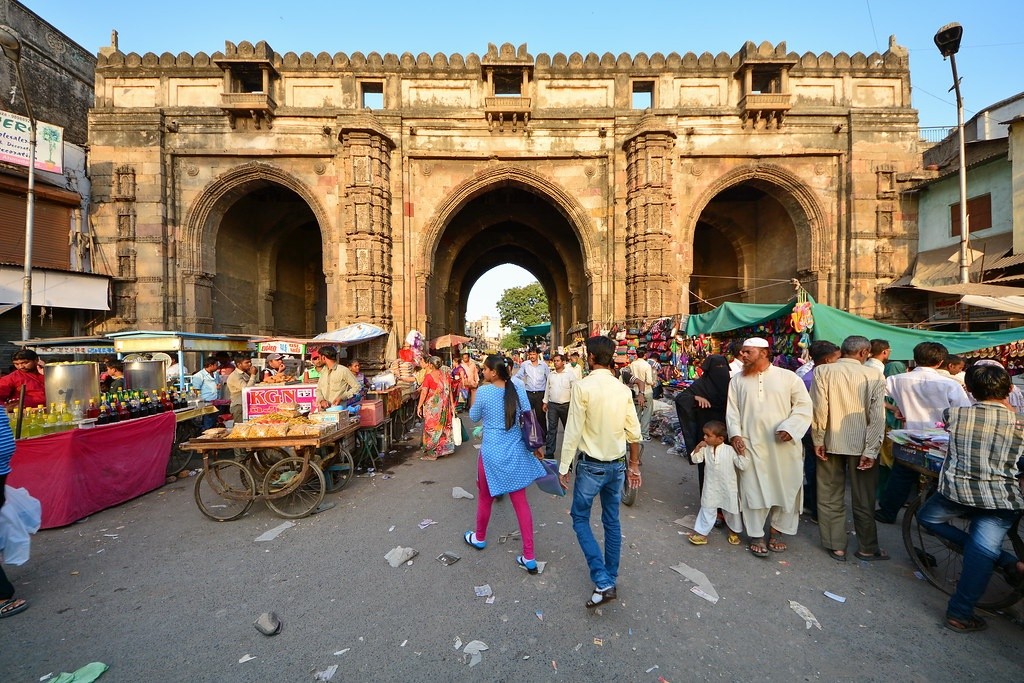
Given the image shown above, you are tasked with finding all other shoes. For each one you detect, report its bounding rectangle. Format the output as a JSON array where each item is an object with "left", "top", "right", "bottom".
[
  {"left": 516, "top": 555, "right": 540, "bottom": 574},
  {"left": 642, "top": 436, "right": 653, "bottom": 442},
  {"left": 464, "top": 529, "right": 488, "bottom": 548},
  {"left": 875, "top": 510, "right": 891, "bottom": 525},
  {"left": 810, "top": 517, "right": 821, "bottom": 525},
  {"left": 585, "top": 585, "right": 618, "bottom": 608},
  {"left": 802, "top": 505, "right": 814, "bottom": 515}
]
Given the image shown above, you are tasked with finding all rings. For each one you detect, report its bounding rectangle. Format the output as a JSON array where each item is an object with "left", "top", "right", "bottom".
[{"left": 737, "top": 441, "right": 739, "bottom": 443}]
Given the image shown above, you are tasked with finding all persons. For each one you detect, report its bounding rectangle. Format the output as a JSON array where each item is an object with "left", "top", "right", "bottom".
[
  {"left": 166, "top": 353, "right": 188, "bottom": 383},
  {"left": 227, "top": 355, "right": 258, "bottom": 463},
  {"left": 797, "top": 336, "right": 891, "bottom": 562},
  {"left": 688, "top": 421, "right": 747, "bottom": 545},
  {"left": 0, "top": 405, "right": 31, "bottom": 618},
  {"left": 558, "top": 335, "right": 645, "bottom": 608},
  {"left": 674, "top": 354, "right": 732, "bottom": 528},
  {"left": 191, "top": 356, "right": 221, "bottom": 431},
  {"left": 630, "top": 347, "right": 661, "bottom": 441},
  {"left": 725, "top": 337, "right": 814, "bottom": 557},
  {"left": 464, "top": 354, "right": 544, "bottom": 576},
  {"left": 418, "top": 347, "right": 585, "bottom": 414},
  {"left": 916, "top": 355, "right": 1024, "bottom": 633},
  {"left": 514, "top": 347, "right": 578, "bottom": 459},
  {"left": 417, "top": 355, "right": 458, "bottom": 460},
  {"left": 874, "top": 341, "right": 973, "bottom": 526},
  {"left": 303, "top": 346, "right": 370, "bottom": 413},
  {"left": 1, "top": 349, "right": 48, "bottom": 413},
  {"left": 260, "top": 353, "right": 286, "bottom": 383},
  {"left": 99, "top": 360, "right": 126, "bottom": 394}
]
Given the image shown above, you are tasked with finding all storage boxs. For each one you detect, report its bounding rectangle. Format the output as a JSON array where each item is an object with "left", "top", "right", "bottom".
[
  {"left": 357, "top": 401, "right": 385, "bottom": 427},
  {"left": 310, "top": 410, "right": 349, "bottom": 428},
  {"left": 366, "top": 386, "right": 403, "bottom": 414},
  {"left": 925, "top": 453, "right": 944, "bottom": 472},
  {"left": 892, "top": 442, "right": 924, "bottom": 464}
]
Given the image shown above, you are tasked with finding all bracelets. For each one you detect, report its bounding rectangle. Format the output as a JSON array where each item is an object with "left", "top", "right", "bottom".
[
  {"left": 630, "top": 459, "right": 639, "bottom": 464},
  {"left": 639, "top": 391, "right": 645, "bottom": 396},
  {"left": 627, "top": 467, "right": 641, "bottom": 476}
]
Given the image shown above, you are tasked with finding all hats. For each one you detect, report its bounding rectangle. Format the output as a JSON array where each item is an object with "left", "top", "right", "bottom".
[
  {"left": 742, "top": 337, "right": 770, "bottom": 348},
  {"left": 265, "top": 352, "right": 288, "bottom": 364},
  {"left": 311, "top": 351, "right": 320, "bottom": 360},
  {"left": 973, "top": 359, "right": 1005, "bottom": 370}
]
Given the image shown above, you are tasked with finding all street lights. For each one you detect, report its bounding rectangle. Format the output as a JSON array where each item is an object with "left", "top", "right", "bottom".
[
  {"left": 0, "top": 24, "right": 38, "bottom": 343},
  {"left": 932, "top": 21, "right": 973, "bottom": 334}
]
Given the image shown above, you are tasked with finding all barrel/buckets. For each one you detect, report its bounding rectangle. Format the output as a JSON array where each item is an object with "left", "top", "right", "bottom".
[
  {"left": 122, "top": 360, "right": 166, "bottom": 392},
  {"left": 44, "top": 362, "right": 101, "bottom": 414}
]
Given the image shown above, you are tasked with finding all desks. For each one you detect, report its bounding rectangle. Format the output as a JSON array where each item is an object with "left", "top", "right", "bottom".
[{"left": 2, "top": 410, "right": 176, "bottom": 531}]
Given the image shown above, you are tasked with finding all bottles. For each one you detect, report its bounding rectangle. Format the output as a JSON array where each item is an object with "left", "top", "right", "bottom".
[
  {"left": 263, "top": 370, "right": 269, "bottom": 379},
  {"left": 304, "top": 368, "right": 309, "bottom": 383},
  {"left": 8, "top": 386, "right": 201, "bottom": 438}
]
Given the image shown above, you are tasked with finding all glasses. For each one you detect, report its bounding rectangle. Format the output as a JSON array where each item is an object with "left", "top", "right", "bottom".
[
  {"left": 866, "top": 349, "right": 873, "bottom": 358},
  {"left": 883, "top": 348, "right": 893, "bottom": 353}
]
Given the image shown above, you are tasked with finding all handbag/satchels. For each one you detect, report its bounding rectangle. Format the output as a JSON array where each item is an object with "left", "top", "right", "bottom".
[
  {"left": 534, "top": 458, "right": 566, "bottom": 496},
  {"left": 520, "top": 407, "right": 549, "bottom": 451},
  {"left": 457, "top": 389, "right": 468, "bottom": 403},
  {"left": 0, "top": 485, "right": 42, "bottom": 566},
  {"left": 451, "top": 410, "right": 470, "bottom": 446}
]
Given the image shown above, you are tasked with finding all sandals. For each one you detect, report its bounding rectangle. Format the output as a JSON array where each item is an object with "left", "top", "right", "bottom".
[
  {"left": 946, "top": 613, "right": 988, "bottom": 634},
  {"left": 690, "top": 512, "right": 787, "bottom": 557},
  {"left": 0, "top": 598, "right": 32, "bottom": 617},
  {"left": 1004, "top": 561, "right": 1024, "bottom": 586}
]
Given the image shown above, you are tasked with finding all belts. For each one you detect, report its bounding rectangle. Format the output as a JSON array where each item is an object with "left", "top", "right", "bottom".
[
  {"left": 577, "top": 452, "right": 623, "bottom": 463},
  {"left": 527, "top": 391, "right": 544, "bottom": 394}
]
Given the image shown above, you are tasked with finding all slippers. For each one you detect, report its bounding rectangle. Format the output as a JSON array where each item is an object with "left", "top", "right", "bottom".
[
  {"left": 828, "top": 549, "right": 846, "bottom": 561},
  {"left": 421, "top": 456, "right": 437, "bottom": 461},
  {"left": 855, "top": 547, "right": 892, "bottom": 561}
]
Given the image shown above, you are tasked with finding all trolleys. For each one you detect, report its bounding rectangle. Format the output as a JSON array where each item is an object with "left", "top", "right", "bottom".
[{"left": 882, "top": 428, "right": 1024, "bottom": 613}]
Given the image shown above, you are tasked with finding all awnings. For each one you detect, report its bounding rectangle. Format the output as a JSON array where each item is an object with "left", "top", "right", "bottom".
[
  {"left": 885, "top": 230, "right": 1024, "bottom": 289},
  {"left": 0, "top": 260, "right": 114, "bottom": 311}
]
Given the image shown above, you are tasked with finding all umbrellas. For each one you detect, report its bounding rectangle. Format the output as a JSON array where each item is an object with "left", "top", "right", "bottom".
[{"left": 428, "top": 333, "right": 472, "bottom": 369}]
